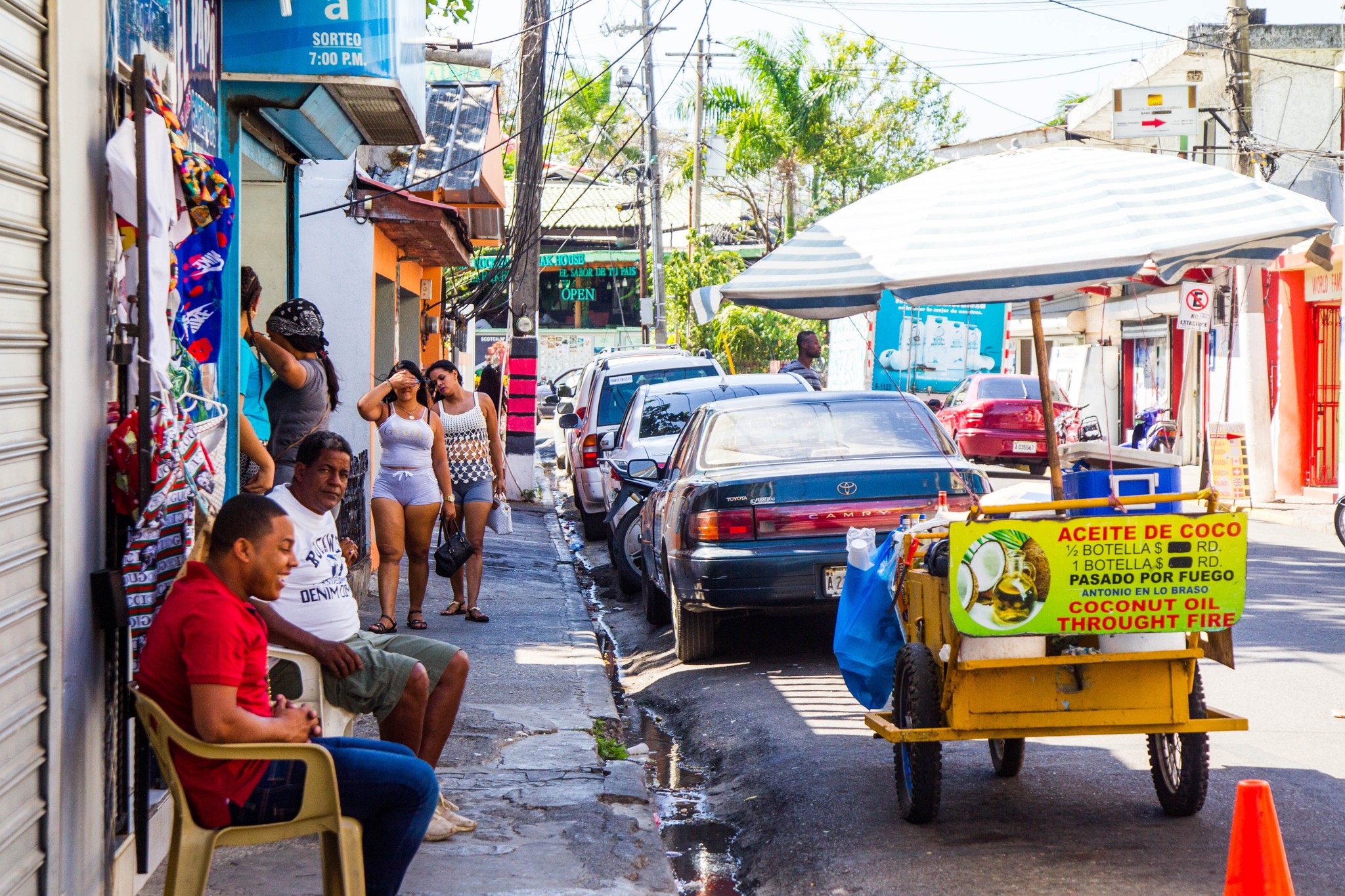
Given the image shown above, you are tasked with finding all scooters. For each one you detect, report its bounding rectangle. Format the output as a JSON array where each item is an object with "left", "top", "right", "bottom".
[
  {"left": 1075, "top": 406, "right": 1103, "bottom": 443},
  {"left": 1118, "top": 408, "right": 1178, "bottom": 454}
]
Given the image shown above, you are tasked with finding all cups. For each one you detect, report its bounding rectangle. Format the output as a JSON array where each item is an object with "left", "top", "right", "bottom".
[{"left": 847, "top": 539, "right": 870, "bottom": 572}]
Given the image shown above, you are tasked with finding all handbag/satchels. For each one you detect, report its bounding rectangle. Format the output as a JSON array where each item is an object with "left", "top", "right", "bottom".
[
  {"left": 485, "top": 492, "right": 513, "bottom": 535},
  {"left": 833, "top": 527, "right": 907, "bottom": 712},
  {"left": 434, "top": 515, "right": 474, "bottom": 578}
]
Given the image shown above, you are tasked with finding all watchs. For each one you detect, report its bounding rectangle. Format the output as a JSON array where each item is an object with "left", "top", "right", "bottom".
[{"left": 443, "top": 494, "right": 456, "bottom": 502}]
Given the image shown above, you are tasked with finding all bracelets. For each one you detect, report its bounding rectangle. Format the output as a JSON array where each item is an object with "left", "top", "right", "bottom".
[{"left": 383, "top": 379, "right": 394, "bottom": 390}]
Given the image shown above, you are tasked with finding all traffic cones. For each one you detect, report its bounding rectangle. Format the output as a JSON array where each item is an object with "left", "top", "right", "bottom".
[{"left": 1219, "top": 777, "right": 1298, "bottom": 896}]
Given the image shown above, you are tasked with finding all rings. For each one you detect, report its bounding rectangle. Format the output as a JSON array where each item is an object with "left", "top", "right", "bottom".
[{"left": 348, "top": 549, "right": 356, "bottom": 554}]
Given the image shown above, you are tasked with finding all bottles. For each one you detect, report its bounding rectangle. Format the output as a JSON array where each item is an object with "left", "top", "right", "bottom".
[
  {"left": 920, "top": 514, "right": 927, "bottom": 523},
  {"left": 992, "top": 549, "right": 1038, "bottom": 625},
  {"left": 899, "top": 515, "right": 903, "bottom": 527},
  {"left": 903, "top": 514, "right": 911, "bottom": 533},
  {"left": 936, "top": 491, "right": 949, "bottom": 512},
  {"left": 910, "top": 513, "right": 920, "bottom": 528}
]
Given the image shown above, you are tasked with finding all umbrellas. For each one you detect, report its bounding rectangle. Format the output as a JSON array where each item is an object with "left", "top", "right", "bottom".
[{"left": 719, "top": 134, "right": 1339, "bottom": 517}]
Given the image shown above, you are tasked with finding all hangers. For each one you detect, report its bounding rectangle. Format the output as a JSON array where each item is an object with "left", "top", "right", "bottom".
[{"left": 174, "top": 366, "right": 228, "bottom": 429}]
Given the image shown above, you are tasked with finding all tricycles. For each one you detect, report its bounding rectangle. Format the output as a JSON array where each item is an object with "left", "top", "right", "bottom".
[{"left": 849, "top": 440, "right": 1252, "bottom": 827}]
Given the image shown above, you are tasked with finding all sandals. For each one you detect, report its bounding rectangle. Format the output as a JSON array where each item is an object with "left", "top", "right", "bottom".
[
  {"left": 441, "top": 601, "right": 467, "bottom": 615},
  {"left": 368, "top": 615, "right": 397, "bottom": 634},
  {"left": 407, "top": 610, "right": 427, "bottom": 630},
  {"left": 465, "top": 607, "right": 489, "bottom": 622}
]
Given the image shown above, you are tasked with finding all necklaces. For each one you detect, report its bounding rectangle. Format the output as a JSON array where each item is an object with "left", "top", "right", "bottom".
[
  {"left": 395, "top": 400, "right": 420, "bottom": 420},
  {"left": 266, "top": 664, "right": 273, "bottom": 701}
]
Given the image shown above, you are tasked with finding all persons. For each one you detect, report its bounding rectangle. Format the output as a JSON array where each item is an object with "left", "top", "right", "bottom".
[
  {"left": 356, "top": 359, "right": 456, "bottom": 634},
  {"left": 248, "top": 429, "right": 471, "bottom": 770},
  {"left": 338, "top": 536, "right": 359, "bottom": 568},
  {"left": 777, "top": 330, "right": 825, "bottom": 392},
  {"left": 240, "top": 264, "right": 345, "bottom": 522},
  {"left": 134, "top": 493, "right": 439, "bottom": 896},
  {"left": 425, "top": 360, "right": 505, "bottom": 622}
]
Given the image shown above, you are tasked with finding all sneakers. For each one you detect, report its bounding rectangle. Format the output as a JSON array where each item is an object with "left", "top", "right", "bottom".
[
  {"left": 436, "top": 791, "right": 478, "bottom": 831},
  {"left": 422, "top": 812, "right": 457, "bottom": 841}
]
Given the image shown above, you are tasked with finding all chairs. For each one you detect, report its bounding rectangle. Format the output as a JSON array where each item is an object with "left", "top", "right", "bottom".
[
  {"left": 126, "top": 679, "right": 368, "bottom": 895},
  {"left": 266, "top": 645, "right": 357, "bottom": 737}
]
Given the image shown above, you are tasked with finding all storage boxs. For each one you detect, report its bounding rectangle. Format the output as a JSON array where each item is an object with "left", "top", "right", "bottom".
[
  {"left": 960, "top": 635, "right": 1046, "bottom": 662},
  {"left": 1057, "top": 442, "right": 1184, "bottom": 517},
  {"left": 1099, "top": 632, "right": 1187, "bottom": 652}
]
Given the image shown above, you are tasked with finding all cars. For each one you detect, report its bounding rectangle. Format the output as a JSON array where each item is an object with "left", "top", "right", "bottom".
[
  {"left": 625, "top": 388, "right": 993, "bottom": 666},
  {"left": 925, "top": 372, "right": 1083, "bottom": 476},
  {"left": 534, "top": 342, "right": 823, "bottom": 580}
]
